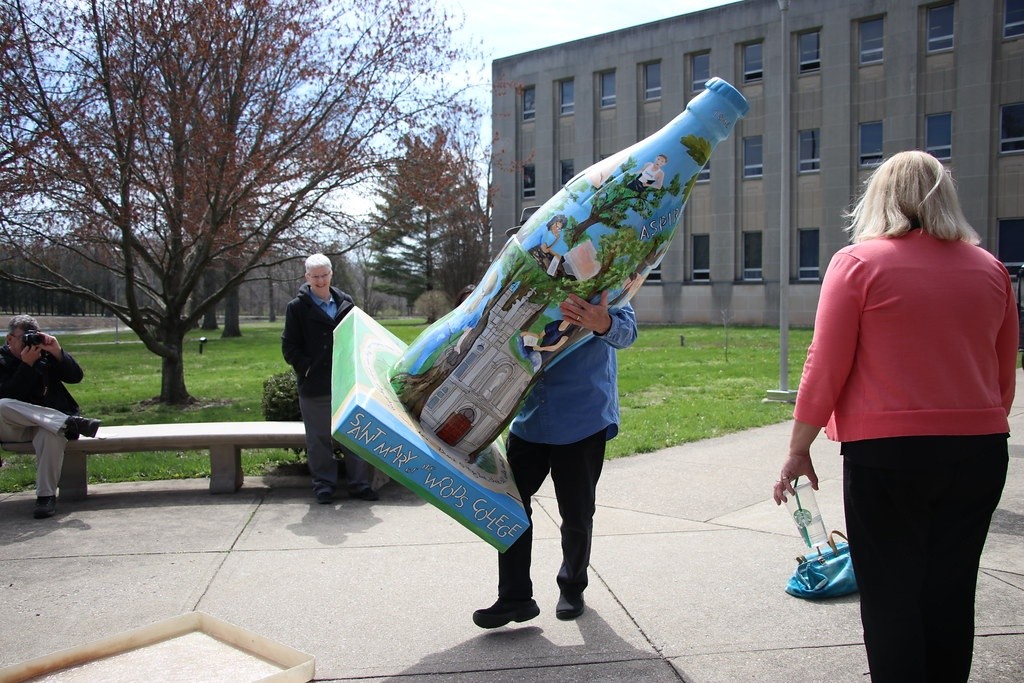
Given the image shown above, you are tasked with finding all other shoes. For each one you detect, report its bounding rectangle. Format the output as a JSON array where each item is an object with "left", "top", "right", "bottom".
[
  {"left": 66, "top": 415, "right": 101, "bottom": 438},
  {"left": 348, "top": 487, "right": 379, "bottom": 501},
  {"left": 472, "top": 596, "right": 540, "bottom": 629},
  {"left": 556, "top": 592, "right": 585, "bottom": 621},
  {"left": 32, "top": 494, "right": 57, "bottom": 519},
  {"left": 318, "top": 492, "right": 333, "bottom": 505}
]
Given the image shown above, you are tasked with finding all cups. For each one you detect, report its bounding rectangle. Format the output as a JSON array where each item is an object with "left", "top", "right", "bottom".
[{"left": 782, "top": 481, "right": 829, "bottom": 548}]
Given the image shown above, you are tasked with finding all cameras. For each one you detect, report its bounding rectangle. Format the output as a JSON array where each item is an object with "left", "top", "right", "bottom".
[{"left": 21, "top": 331, "right": 44, "bottom": 348}]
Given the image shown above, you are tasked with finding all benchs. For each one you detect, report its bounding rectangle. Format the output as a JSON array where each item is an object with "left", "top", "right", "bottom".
[{"left": 0, "top": 420, "right": 390, "bottom": 503}]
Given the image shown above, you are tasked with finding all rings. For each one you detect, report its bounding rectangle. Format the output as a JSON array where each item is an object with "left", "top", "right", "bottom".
[
  {"left": 783, "top": 477, "right": 789, "bottom": 480},
  {"left": 577, "top": 316, "right": 579, "bottom": 320},
  {"left": 777, "top": 481, "right": 782, "bottom": 482}
]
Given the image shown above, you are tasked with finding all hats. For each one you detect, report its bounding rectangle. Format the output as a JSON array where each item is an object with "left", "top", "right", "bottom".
[{"left": 505, "top": 206, "right": 540, "bottom": 238}]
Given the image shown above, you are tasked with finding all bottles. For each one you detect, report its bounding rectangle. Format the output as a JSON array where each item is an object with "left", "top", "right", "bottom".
[{"left": 390, "top": 76, "right": 749, "bottom": 458}]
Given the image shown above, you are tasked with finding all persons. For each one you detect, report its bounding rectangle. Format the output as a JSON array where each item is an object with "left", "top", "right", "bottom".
[
  {"left": 0, "top": 314, "right": 101, "bottom": 519},
  {"left": 473, "top": 205, "right": 637, "bottom": 629},
  {"left": 774, "top": 150, "right": 1019, "bottom": 683},
  {"left": 281, "top": 253, "right": 378, "bottom": 504}
]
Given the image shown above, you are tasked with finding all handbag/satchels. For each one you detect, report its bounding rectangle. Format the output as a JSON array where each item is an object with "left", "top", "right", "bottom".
[{"left": 783, "top": 531, "right": 858, "bottom": 598}]
